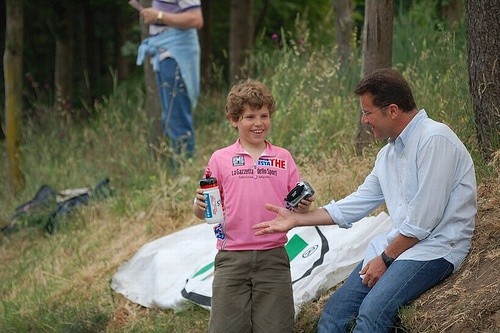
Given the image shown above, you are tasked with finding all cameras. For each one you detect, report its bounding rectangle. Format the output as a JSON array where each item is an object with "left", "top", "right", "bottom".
[{"left": 284, "top": 181, "right": 315, "bottom": 209}]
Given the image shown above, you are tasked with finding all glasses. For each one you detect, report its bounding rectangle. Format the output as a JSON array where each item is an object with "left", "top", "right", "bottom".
[{"left": 362, "top": 103, "right": 391, "bottom": 118}]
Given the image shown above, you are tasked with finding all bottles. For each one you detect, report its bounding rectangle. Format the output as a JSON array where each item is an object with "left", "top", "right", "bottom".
[{"left": 199, "top": 168, "right": 225, "bottom": 224}]
